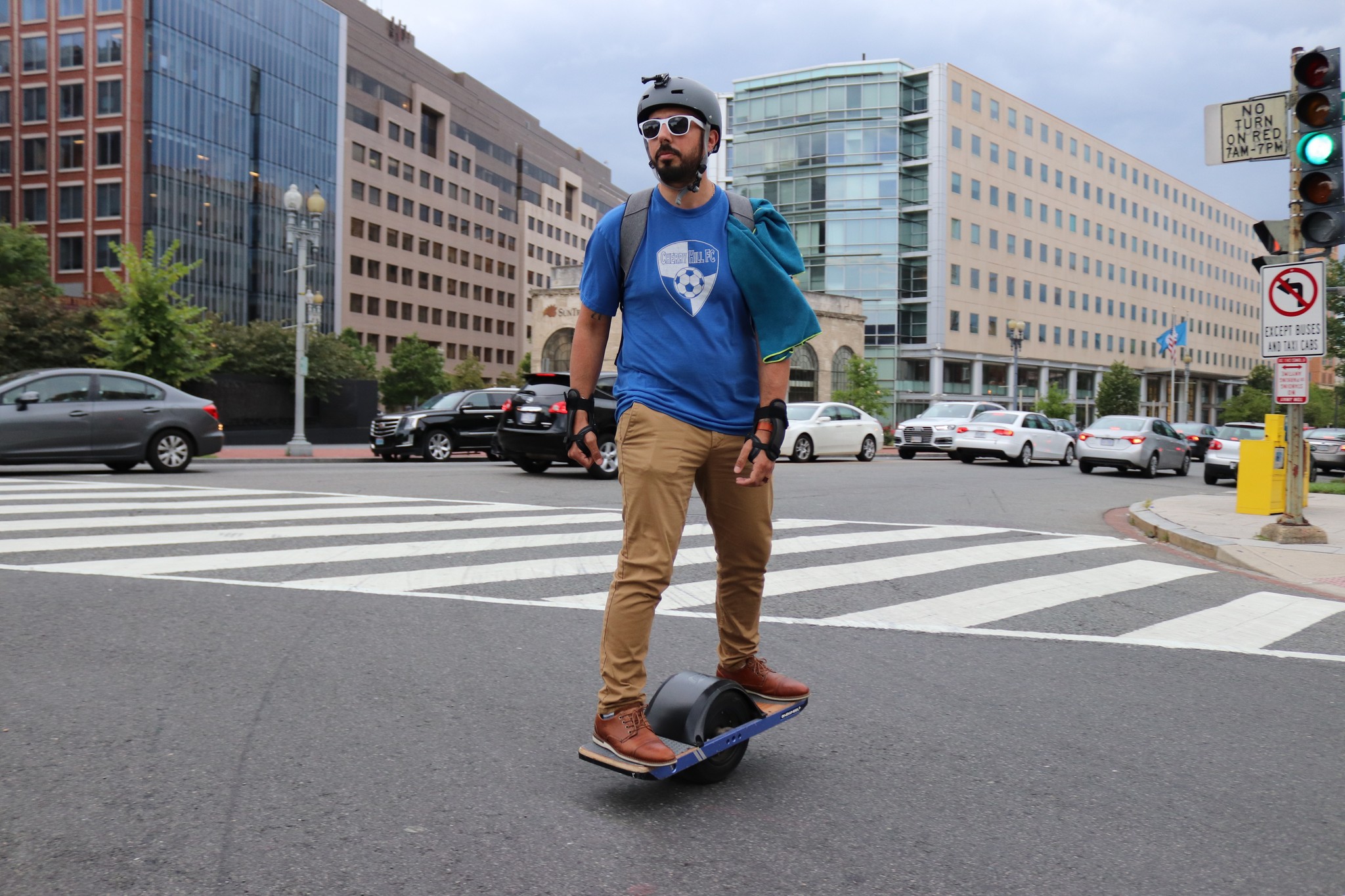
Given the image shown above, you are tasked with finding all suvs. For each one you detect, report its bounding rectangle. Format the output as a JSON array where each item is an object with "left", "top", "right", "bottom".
[
  {"left": 369, "top": 387, "right": 521, "bottom": 463},
  {"left": 1203, "top": 422, "right": 1318, "bottom": 486},
  {"left": 488, "top": 372, "right": 626, "bottom": 480},
  {"left": 893, "top": 401, "right": 1007, "bottom": 460}
]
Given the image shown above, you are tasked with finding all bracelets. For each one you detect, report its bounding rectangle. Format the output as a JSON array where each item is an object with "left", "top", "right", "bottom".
[{"left": 757, "top": 422, "right": 773, "bottom": 432}]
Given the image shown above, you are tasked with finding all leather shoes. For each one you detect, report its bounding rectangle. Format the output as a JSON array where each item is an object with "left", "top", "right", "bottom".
[
  {"left": 717, "top": 654, "right": 810, "bottom": 703},
  {"left": 591, "top": 707, "right": 678, "bottom": 767}
]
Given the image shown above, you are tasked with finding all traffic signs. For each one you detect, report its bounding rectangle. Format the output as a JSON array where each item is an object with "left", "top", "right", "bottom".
[{"left": 1274, "top": 357, "right": 1310, "bottom": 405}]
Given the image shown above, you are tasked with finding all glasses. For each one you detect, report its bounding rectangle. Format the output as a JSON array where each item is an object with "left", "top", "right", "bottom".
[{"left": 638, "top": 114, "right": 705, "bottom": 139}]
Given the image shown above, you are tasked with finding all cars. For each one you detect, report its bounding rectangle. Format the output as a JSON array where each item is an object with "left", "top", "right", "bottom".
[
  {"left": 1048, "top": 417, "right": 1084, "bottom": 442},
  {"left": 1302, "top": 425, "right": 1345, "bottom": 476},
  {"left": 950, "top": 410, "right": 1076, "bottom": 467},
  {"left": 0, "top": 368, "right": 226, "bottom": 472},
  {"left": 777, "top": 401, "right": 885, "bottom": 461},
  {"left": 1166, "top": 421, "right": 1220, "bottom": 464},
  {"left": 1077, "top": 414, "right": 1192, "bottom": 479}
]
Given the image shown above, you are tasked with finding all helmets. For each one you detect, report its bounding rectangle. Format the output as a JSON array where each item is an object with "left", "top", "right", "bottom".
[{"left": 637, "top": 76, "right": 721, "bottom": 155}]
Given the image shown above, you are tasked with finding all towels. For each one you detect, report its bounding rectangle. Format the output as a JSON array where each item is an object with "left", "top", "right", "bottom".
[{"left": 726, "top": 197, "right": 822, "bottom": 366}]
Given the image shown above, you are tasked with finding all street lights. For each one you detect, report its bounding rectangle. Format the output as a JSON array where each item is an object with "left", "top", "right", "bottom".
[
  {"left": 282, "top": 184, "right": 326, "bottom": 457},
  {"left": 1183, "top": 354, "right": 1192, "bottom": 423},
  {"left": 1006, "top": 317, "right": 1025, "bottom": 410}
]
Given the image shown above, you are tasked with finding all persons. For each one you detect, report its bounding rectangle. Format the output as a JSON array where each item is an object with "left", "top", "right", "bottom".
[{"left": 565, "top": 76, "right": 821, "bottom": 766}]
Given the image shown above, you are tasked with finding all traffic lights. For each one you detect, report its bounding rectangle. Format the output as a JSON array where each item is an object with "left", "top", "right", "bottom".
[
  {"left": 1251, "top": 218, "right": 1294, "bottom": 275},
  {"left": 1292, "top": 47, "right": 1345, "bottom": 251}
]
